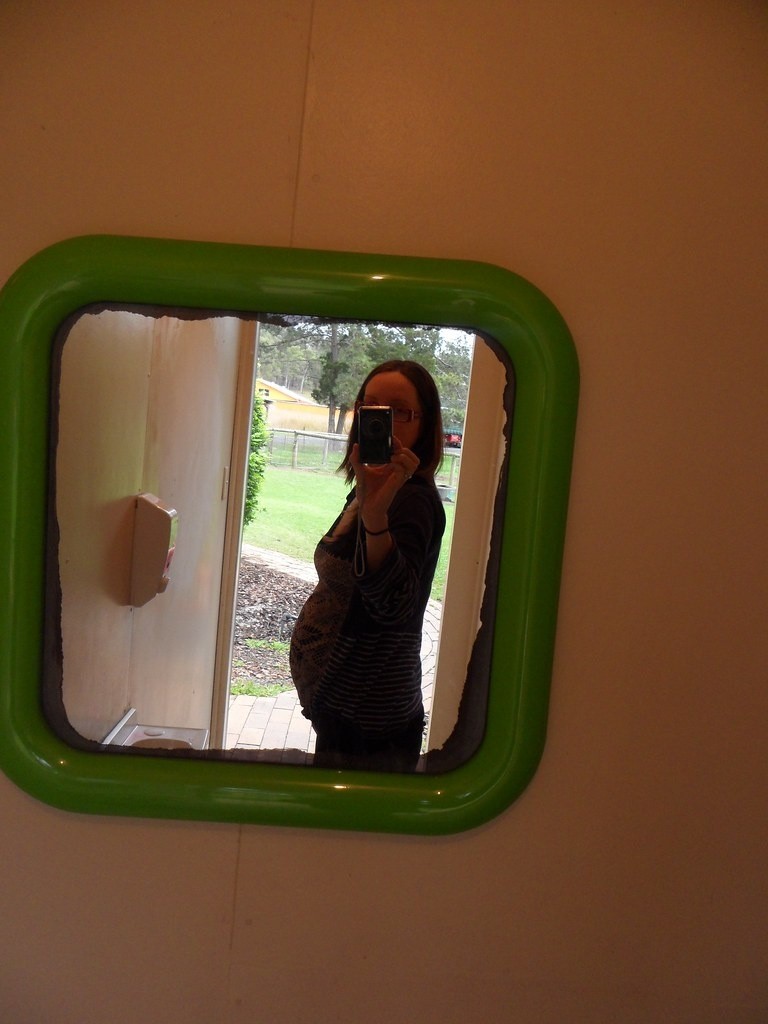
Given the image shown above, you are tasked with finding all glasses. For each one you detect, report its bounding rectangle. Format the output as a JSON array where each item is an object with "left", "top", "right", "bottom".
[{"left": 355, "top": 400, "right": 422, "bottom": 424}]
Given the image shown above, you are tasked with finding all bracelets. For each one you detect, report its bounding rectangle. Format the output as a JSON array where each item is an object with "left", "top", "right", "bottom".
[{"left": 363, "top": 525, "right": 390, "bottom": 535}]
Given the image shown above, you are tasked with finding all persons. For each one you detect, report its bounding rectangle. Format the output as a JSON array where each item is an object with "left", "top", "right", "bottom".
[{"left": 288, "top": 360, "right": 444, "bottom": 772}]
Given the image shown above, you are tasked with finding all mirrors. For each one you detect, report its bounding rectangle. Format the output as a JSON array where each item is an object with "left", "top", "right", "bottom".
[{"left": 0, "top": 233, "right": 580, "bottom": 840}]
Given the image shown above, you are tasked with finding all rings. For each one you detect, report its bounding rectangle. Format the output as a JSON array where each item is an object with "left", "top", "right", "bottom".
[{"left": 405, "top": 474, "right": 413, "bottom": 479}]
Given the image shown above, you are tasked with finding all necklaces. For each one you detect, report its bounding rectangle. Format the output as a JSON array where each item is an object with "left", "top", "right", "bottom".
[{"left": 345, "top": 505, "right": 359, "bottom": 512}]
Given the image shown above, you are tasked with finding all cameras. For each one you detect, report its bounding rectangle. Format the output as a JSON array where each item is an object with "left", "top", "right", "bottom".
[{"left": 357, "top": 405, "right": 394, "bottom": 464}]
